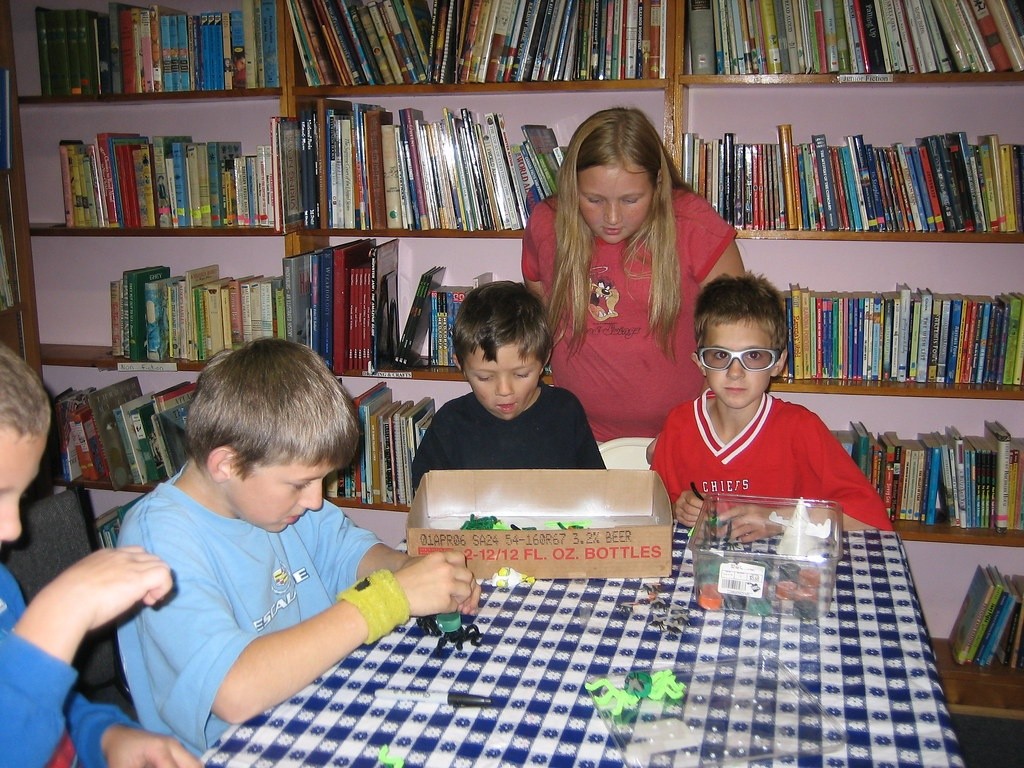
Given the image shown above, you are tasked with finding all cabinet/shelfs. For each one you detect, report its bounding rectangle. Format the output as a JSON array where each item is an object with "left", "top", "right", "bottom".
[
  {"left": 672, "top": 0, "right": 1024, "bottom": 721},
  {"left": 283, "top": 0, "right": 670, "bottom": 489},
  {"left": 0, "top": 0, "right": 291, "bottom": 493}
]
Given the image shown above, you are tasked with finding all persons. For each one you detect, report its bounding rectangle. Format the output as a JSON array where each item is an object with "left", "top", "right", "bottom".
[
  {"left": 0, "top": 345, "right": 205, "bottom": 768},
  {"left": 416, "top": 280, "right": 606, "bottom": 497},
  {"left": 520, "top": 106, "right": 745, "bottom": 463},
  {"left": 649, "top": 274, "right": 894, "bottom": 544},
  {"left": 116, "top": 338, "right": 482, "bottom": 759}
]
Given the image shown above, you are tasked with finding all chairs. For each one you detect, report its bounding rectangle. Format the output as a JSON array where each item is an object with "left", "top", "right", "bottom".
[{"left": 0, "top": 482, "right": 139, "bottom": 725}]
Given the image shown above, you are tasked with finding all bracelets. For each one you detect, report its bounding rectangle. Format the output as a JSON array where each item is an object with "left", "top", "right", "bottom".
[{"left": 336, "top": 569, "right": 410, "bottom": 645}]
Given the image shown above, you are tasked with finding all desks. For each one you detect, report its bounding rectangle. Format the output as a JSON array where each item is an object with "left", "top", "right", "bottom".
[{"left": 197, "top": 517, "right": 969, "bottom": 768}]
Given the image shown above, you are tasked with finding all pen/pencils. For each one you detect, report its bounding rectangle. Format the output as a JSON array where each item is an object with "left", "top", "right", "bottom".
[{"left": 375, "top": 689, "right": 494, "bottom": 707}]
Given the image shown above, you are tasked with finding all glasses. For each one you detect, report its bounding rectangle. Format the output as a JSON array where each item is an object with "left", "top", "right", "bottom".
[{"left": 698, "top": 347, "right": 780, "bottom": 372}]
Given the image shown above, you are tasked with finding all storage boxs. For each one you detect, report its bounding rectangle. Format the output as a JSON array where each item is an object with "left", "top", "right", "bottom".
[
  {"left": 405, "top": 468, "right": 672, "bottom": 581},
  {"left": 687, "top": 490, "right": 842, "bottom": 618}
]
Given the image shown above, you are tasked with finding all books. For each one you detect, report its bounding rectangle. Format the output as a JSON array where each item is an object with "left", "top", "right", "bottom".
[
  {"left": 270, "top": 97, "right": 568, "bottom": 235},
  {"left": 326, "top": 381, "right": 435, "bottom": 507},
  {"left": 110, "top": 264, "right": 286, "bottom": 362},
  {"left": 34, "top": 0, "right": 280, "bottom": 96},
  {"left": 948, "top": 563, "right": 1024, "bottom": 669},
  {"left": 54, "top": 376, "right": 197, "bottom": 493},
  {"left": 830, "top": 420, "right": 1024, "bottom": 535},
  {"left": 60, "top": 133, "right": 271, "bottom": 227},
  {"left": 95, "top": 494, "right": 147, "bottom": 549},
  {"left": 686, "top": 0, "right": 1024, "bottom": 75},
  {"left": 777, "top": 282, "right": 1024, "bottom": 385},
  {"left": 286, "top": 0, "right": 667, "bottom": 88},
  {"left": 285, "top": 238, "right": 494, "bottom": 375},
  {"left": 682, "top": 124, "right": 1024, "bottom": 232}
]
